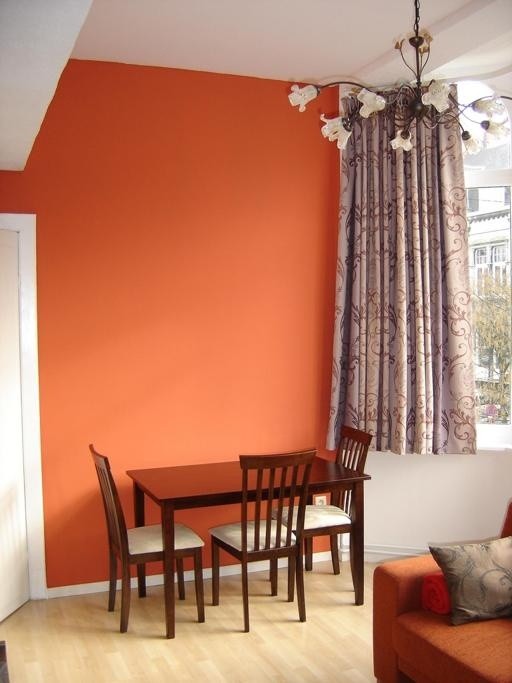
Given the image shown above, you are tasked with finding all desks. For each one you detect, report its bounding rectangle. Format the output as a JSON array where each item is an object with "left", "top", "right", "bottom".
[{"left": 127, "top": 455, "right": 372, "bottom": 638}]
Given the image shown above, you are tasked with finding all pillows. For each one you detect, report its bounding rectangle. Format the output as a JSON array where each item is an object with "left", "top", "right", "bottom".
[
  {"left": 421, "top": 570, "right": 452, "bottom": 615},
  {"left": 429, "top": 536, "right": 512, "bottom": 625}
]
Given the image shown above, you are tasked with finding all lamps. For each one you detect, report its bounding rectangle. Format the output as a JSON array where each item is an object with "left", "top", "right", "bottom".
[{"left": 289, "top": 0, "right": 512, "bottom": 154}]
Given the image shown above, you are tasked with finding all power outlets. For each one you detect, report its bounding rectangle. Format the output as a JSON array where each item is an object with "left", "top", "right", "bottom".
[{"left": 315, "top": 496, "right": 326, "bottom": 505}]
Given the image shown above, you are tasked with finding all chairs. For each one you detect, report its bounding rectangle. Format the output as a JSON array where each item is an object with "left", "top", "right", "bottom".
[
  {"left": 89, "top": 444, "right": 205, "bottom": 632},
  {"left": 272, "top": 425, "right": 373, "bottom": 575},
  {"left": 373, "top": 502, "right": 512, "bottom": 683},
  {"left": 208, "top": 447, "right": 318, "bottom": 632}
]
